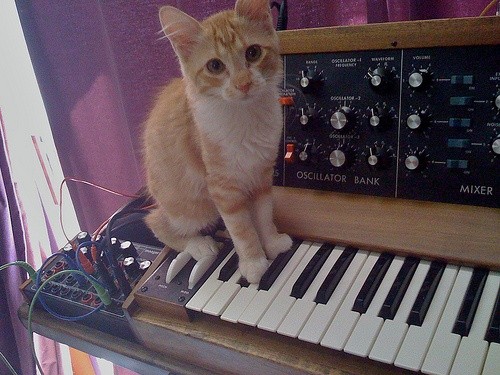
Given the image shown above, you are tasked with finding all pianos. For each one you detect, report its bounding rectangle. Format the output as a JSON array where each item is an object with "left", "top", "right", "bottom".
[{"left": 15, "top": 15, "right": 500, "bottom": 374}]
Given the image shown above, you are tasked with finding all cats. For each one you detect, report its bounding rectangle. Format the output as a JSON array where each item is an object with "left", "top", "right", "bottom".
[{"left": 142, "top": 0, "right": 293, "bottom": 285}]
